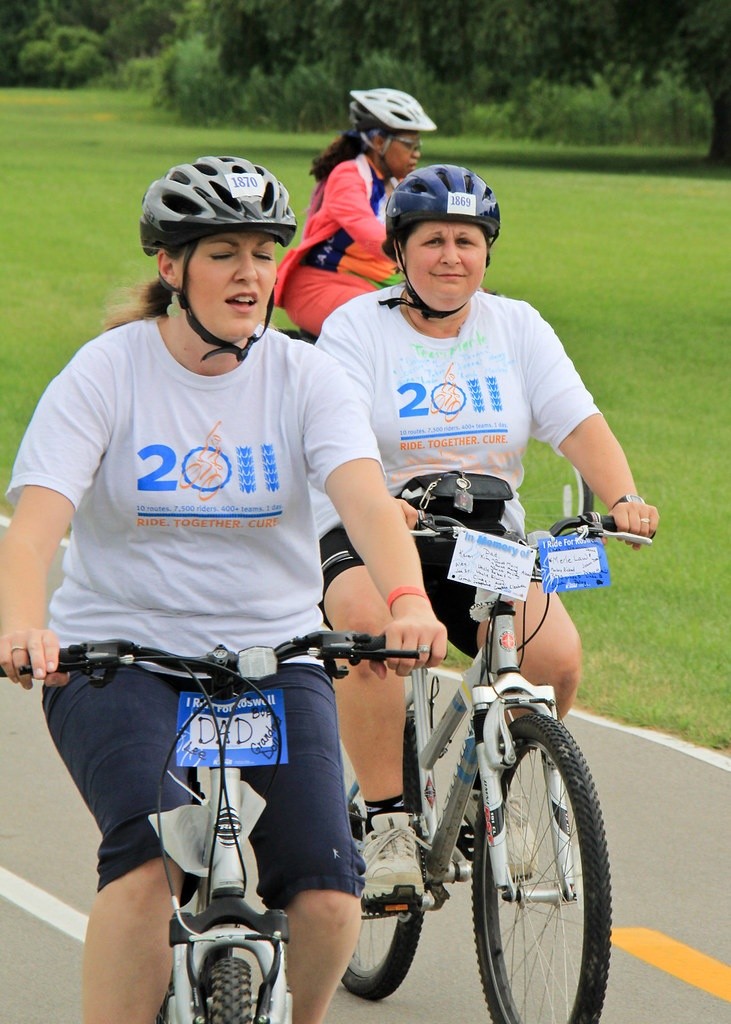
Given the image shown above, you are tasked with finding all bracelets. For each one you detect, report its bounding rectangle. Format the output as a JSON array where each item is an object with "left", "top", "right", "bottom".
[{"left": 385, "top": 585, "right": 432, "bottom": 617}]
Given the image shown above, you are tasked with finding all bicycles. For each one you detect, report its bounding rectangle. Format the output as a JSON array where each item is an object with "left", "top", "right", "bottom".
[
  {"left": 0, "top": 629, "right": 451, "bottom": 1024},
  {"left": 322, "top": 510, "right": 655, "bottom": 1023}
]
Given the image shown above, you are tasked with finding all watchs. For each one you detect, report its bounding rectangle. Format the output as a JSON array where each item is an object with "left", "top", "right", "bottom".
[{"left": 611, "top": 493, "right": 649, "bottom": 510}]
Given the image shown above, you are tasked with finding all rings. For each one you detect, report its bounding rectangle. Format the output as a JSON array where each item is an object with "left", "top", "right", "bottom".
[
  {"left": 640, "top": 517, "right": 651, "bottom": 525},
  {"left": 416, "top": 643, "right": 432, "bottom": 653},
  {"left": 10, "top": 645, "right": 28, "bottom": 654}
]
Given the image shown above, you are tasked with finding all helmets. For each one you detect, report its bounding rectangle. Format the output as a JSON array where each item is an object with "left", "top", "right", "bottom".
[
  {"left": 350, "top": 85, "right": 440, "bottom": 134},
  {"left": 139, "top": 152, "right": 299, "bottom": 256},
  {"left": 381, "top": 164, "right": 501, "bottom": 242}
]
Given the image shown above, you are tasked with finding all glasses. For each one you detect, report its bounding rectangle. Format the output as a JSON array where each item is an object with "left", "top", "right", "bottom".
[{"left": 382, "top": 135, "right": 426, "bottom": 152}]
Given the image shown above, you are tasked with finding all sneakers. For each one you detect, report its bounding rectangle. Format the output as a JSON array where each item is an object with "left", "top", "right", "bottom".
[
  {"left": 359, "top": 811, "right": 426, "bottom": 911},
  {"left": 458, "top": 775, "right": 540, "bottom": 883}
]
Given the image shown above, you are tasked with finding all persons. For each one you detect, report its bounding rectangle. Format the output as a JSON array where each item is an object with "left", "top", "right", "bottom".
[
  {"left": 284, "top": 157, "right": 665, "bottom": 930},
  {"left": 2, "top": 153, "right": 452, "bottom": 1024},
  {"left": 269, "top": 84, "right": 440, "bottom": 341}
]
